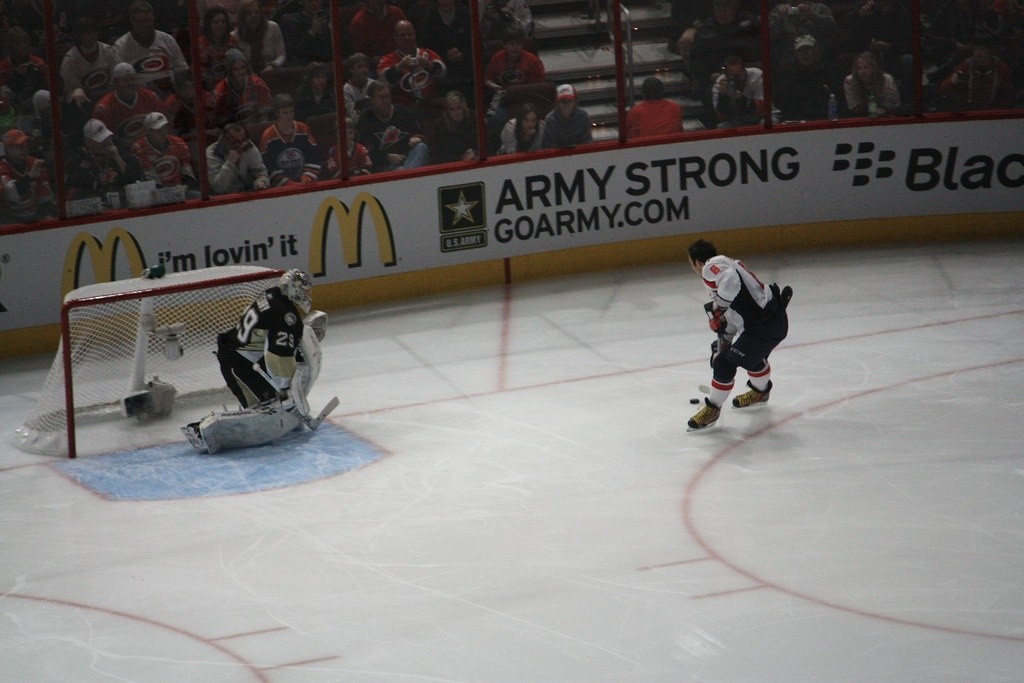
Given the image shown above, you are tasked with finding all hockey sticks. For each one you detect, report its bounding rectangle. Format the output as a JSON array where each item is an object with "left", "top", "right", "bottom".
[
  {"left": 252, "top": 362, "right": 340, "bottom": 431},
  {"left": 710, "top": 330, "right": 724, "bottom": 368}
]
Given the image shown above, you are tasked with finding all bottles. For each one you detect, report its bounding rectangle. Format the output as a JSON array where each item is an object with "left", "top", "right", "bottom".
[{"left": 828, "top": 93, "right": 838, "bottom": 121}]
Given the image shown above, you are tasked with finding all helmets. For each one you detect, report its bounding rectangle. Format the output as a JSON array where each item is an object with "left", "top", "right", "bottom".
[{"left": 279, "top": 270, "right": 312, "bottom": 313}]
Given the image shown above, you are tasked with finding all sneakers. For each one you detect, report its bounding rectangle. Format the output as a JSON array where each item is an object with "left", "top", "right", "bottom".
[
  {"left": 685, "top": 397, "right": 722, "bottom": 431},
  {"left": 731, "top": 379, "right": 772, "bottom": 410}
]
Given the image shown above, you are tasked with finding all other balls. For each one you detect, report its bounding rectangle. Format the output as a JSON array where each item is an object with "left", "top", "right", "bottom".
[{"left": 690, "top": 399, "right": 699, "bottom": 404}]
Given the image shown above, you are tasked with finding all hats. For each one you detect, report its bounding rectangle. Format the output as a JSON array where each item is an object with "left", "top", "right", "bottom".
[
  {"left": 3, "top": 129, "right": 30, "bottom": 144},
  {"left": 73, "top": 17, "right": 99, "bottom": 36},
  {"left": 795, "top": 35, "right": 817, "bottom": 52},
  {"left": 555, "top": 83, "right": 577, "bottom": 104},
  {"left": 113, "top": 62, "right": 136, "bottom": 78},
  {"left": 224, "top": 49, "right": 252, "bottom": 81},
  {"left": 143, "top": 112, "right": 169, "bottom": 130},
  {"left": 83, "top": 119, "right": 114, "bottom": 142},
  {"left": 33, "top": 90, "right": 51, "bottom": 118}
]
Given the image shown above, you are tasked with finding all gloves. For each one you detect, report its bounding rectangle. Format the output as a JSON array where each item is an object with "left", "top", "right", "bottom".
[
  {"left": 710, "top": 309, "right": 728, "bottom": 333},
  {"left": 720, "top": 338, "right": 732, "bottom": 353}
]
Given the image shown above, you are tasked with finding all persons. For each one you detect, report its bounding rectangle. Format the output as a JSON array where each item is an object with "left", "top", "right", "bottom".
[
  {"left": 667, "top": 0, "right": 1024, "bottom": 130},
  {"left": 0, "top": 0, "right": 548, "bottom": 225},
  {"left": 543, "top": 84, "right": 591, "bottom": 149},
  {"left": 625, "top": 77, "right": 682, "bottom": 136},
  {"left": 687, "top": 240, "right": 789, "bottom": 432},
  {"left": 216, "top": 266, "right": 314, "bottom": 407}
]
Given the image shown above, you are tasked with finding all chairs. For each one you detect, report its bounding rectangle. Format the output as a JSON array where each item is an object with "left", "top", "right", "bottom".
[{"left": 168, "top": 0, "right": 559, "bottom": 157}]
